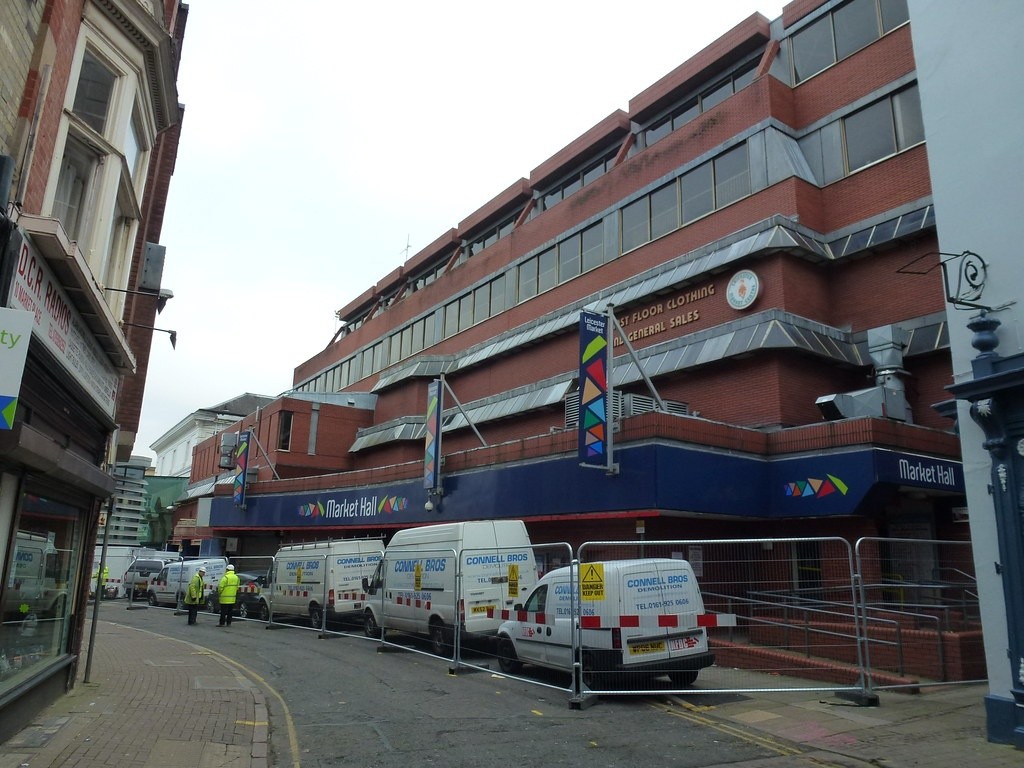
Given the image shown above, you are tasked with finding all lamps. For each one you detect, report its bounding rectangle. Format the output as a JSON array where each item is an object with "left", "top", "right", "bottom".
[
  {"left": 100, "top": 283, "right": 169, "bottom": 316},
  {"left": 119, "top": 319, "right": 177, "bottom": 351},
  {"left": 348, "top": 399, "right": 356, "bottom": 405},
  {"left": 424, "top": 496, "right": 434, "bottom": 512}
]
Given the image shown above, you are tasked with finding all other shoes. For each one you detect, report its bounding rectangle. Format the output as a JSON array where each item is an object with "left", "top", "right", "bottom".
[
  {"left": 227, "top": 624, "right": 231, "bottom": 628},
  {"left": 216, "top": 623, "right": 225, "bottom": 627}
]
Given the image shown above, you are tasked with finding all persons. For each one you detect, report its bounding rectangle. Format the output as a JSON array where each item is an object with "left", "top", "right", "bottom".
[
  {"left": 92, "top": 561, "right": 109, "bottom": 601},
  {"left": 216, "top": 565, "right": 240, "bottom": 627},
  {"left": 183, "top": 566, "right": 206, "bottom": 626}
]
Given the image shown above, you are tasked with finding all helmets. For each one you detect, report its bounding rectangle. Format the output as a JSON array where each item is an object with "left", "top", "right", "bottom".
[
  {"left": 226, "top": 565, "right": 235, "bottom": 571},
  {"left": 198, "top": 567, "right": 207, "bottom": 574}
]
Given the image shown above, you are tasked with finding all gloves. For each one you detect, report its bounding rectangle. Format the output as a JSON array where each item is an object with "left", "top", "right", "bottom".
[{"left": 192, "top": 598, "right": 197, "bottom": 602}]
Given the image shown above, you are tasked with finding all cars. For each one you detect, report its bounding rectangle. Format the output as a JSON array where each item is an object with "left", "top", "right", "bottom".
[{"left": 207, "top": 569, "right": 269, "bottom": 618}]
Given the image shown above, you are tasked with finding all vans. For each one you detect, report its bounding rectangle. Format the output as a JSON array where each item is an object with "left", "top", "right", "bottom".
[
  {"left": 359, "top": 520, "right": 540, "bottom": 661},
  {"left": 88, "top": 546, "right": 179, "bottom": 600},
  {"left": 146, "top": 558, "right": 228, "bottom": 610},
  {"left": 256, "top": 535, "right": 385, "bottom": 630},
  {"left": 2, "top": 529, "right": 70, "bottom": 634},
  {"left": 495, "top": 557, "right": 717, "bottom": 700}
]
